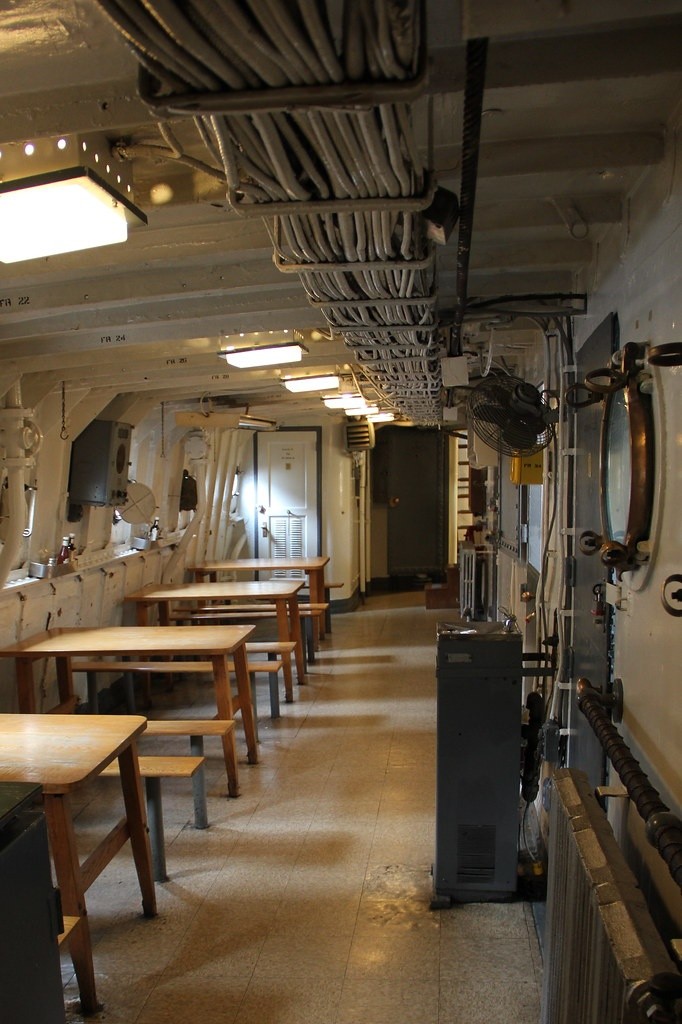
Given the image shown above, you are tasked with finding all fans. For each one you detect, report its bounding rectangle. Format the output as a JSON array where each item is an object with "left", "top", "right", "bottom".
[{"left": 465, "top": 374, "right": 565, "bottom": 458}]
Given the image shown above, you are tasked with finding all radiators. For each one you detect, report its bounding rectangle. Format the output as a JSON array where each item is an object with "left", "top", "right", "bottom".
[{"left": 540, "top": 768, "right": 682, "bottom": 1024}]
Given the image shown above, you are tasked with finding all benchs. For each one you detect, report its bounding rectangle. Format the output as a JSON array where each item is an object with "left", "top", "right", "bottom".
[{"left": 55, "top": 583, "right": 348, "bottom": 967}]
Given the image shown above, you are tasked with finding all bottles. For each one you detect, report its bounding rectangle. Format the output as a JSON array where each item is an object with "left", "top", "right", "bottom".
[
  {"left": 142, "top": 516, "right": 165, "bottom": 542},
  {"left": 40, "top": 533, "right": 75, "bottom": 566}
]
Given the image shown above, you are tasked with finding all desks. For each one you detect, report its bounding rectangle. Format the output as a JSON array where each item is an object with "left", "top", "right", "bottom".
[
  {"left": 184, "top": 557, "right": 331, "bottom": 651},
  {"left": 0, "top": 625, "right": 258, "bottom": 798},
  {"left": 0, "top": 712, "right": 159, "bottom": 1007},
  {"left": 125, "top": 581, "right": 306, "bottom": 716}
]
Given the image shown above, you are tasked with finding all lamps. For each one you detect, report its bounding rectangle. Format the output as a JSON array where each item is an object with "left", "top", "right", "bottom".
[
  {"left": 0, "top": 130, "right": 149, "bottom": 264},
  {"left": 278, "top": 375, "right": 344, "bottom": 393},
  {"left": 319, "top": 395, "right": 395, "bottom": 423},
  {"left": 216, "top": 329, "right": 308, "bottom": 371}
]
